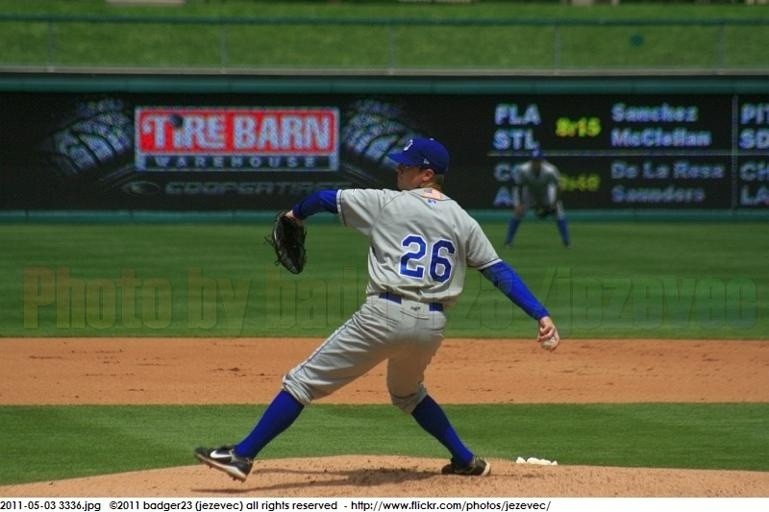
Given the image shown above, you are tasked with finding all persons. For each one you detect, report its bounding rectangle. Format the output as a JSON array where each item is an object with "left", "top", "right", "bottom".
[
  {"left": 505, "top": 150, "right": 570, "bottom": 250},
  {"left": 194, "top": 138, "right": 562, "bottom": 481}
]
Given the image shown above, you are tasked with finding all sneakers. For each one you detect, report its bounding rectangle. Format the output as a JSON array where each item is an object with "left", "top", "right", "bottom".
[
  {"left": 192, "top": 445, "right": 256, "bottom": 483},
  {"left": 440, "top": 456, "right": 493, "bottom": 478}
]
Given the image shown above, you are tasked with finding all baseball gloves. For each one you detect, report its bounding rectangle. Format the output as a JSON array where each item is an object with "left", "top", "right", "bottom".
[
  {"left": 272, "top": 209, "right": 306, "bottom": 274},
  {"left": 536, "top": 201, "right": 556, "bottom": 218}
]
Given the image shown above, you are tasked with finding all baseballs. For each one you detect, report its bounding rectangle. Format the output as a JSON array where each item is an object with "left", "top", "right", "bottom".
[{"left": 540, "top": 333, "right": 557, "bottom": 351}]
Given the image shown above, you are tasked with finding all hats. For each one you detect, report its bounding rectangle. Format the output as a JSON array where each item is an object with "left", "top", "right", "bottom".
[
  {"left": 383, "top": 135, "right": 451, "bottom": 173},
  {"left": 529, "top": 149, "right": 543, "bottom": 159}
]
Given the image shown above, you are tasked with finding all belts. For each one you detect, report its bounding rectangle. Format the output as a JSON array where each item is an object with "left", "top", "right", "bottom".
[{"left": 378, "top": 292, "right": 444, "bottom": 313}]
